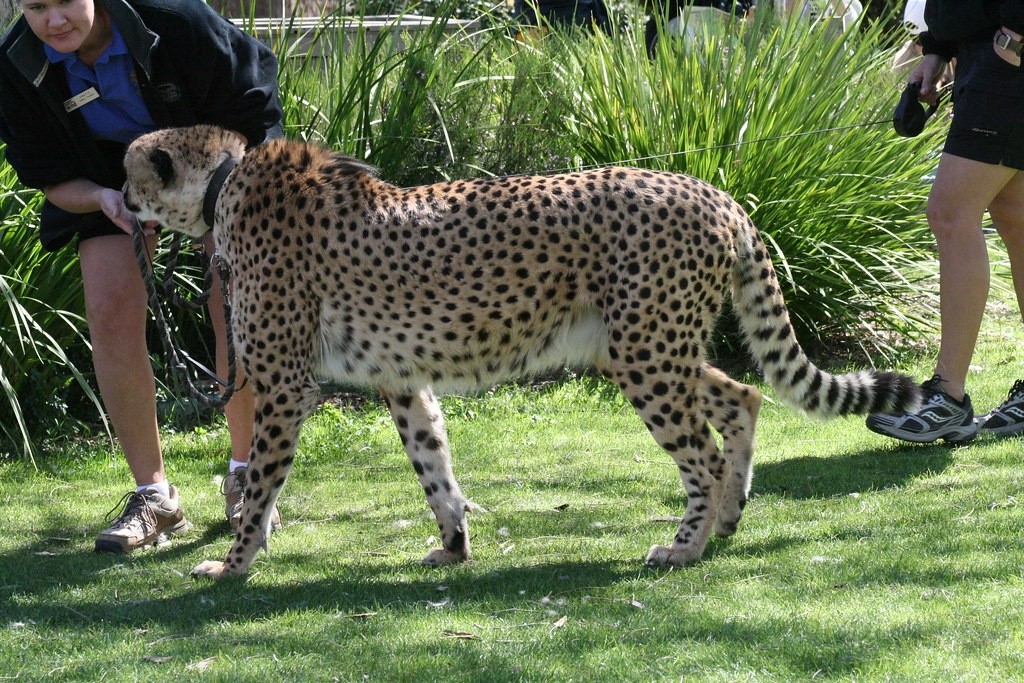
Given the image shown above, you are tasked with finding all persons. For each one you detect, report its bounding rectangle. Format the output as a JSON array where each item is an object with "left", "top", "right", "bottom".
[
  {"left": 509, "top": 1, "right": 955, "bottom": 86},
  {"left": 864, "top": 1, "right": 1024, "bottom": 441},
  {"left": 0, "top": 0, "right": 281, "bottom": 550}
]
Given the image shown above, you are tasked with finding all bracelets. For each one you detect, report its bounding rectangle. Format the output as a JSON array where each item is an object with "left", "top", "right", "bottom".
[{"left": 993, "top": 28, "right": 1023, "bottom": 57}]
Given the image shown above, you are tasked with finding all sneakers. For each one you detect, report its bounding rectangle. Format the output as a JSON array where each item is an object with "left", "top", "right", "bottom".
[
  {"left": 94, "top": 486, "right": 189, "bottom": 552},
  {"left": 974, "top": 378, "right": 1024, "bottom": 433},
  {"left": 220, "top": 466, "right": 281, "bottom": 531},
  {"left": 866, "top": 374, "right": 977, "bottom": 443}
]
